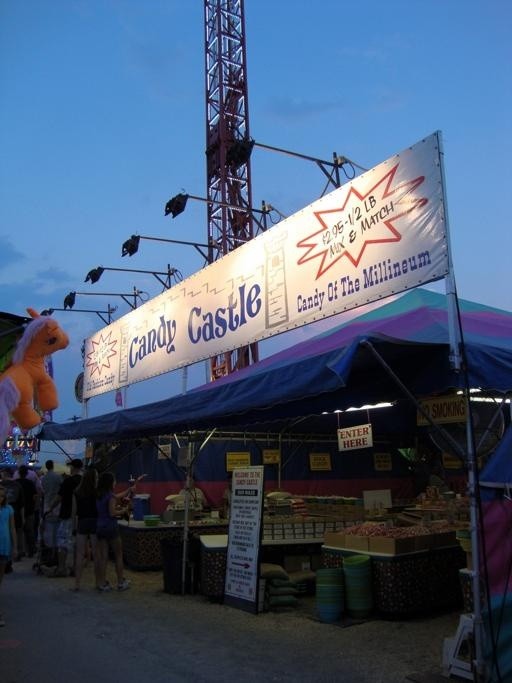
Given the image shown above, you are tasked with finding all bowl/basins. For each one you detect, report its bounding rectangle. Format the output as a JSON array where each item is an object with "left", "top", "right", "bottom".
[
  {"left": 311, "top": 568, "right": 346, "bottom": 623},
  {"left": 453, "top": 537, "right": 472, "bottom": 552},
  {"left": 440, "top": 491, "right": 455, "bottom": 498},
  {"left": 342, "top": 554, "right": 376, "bottom": 618},
  {"left": 144, "top": 515, "right": 160, "bottom": 526}
]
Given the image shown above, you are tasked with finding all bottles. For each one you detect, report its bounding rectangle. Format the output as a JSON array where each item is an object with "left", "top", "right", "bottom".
[{"left": 129, "top": 512, "right": 134, "bottom": 526}]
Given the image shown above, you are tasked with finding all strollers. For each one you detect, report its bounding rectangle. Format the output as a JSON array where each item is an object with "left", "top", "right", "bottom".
[{"left": 30, "top": 509, "right": 73, "bottom": 576}]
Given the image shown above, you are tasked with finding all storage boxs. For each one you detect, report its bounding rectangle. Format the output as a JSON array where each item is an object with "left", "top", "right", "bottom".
[{"left": 323, "top": 527, "right": 456, "bottom": 554}]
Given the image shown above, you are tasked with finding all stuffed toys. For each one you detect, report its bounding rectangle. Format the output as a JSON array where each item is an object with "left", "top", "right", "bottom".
[{"left": 0, "top": 306, "right": 70, "bottom": 436}]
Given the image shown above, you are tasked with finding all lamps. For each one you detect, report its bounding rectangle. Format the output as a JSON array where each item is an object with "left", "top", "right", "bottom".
[
  {"left": 216, "top": 139, "right": 342, "bottom": 189},
  {"left": 61, "top": 286, "right": 141, "bottom": 308},
  {"left": 39, "top": 302, "right": 112, "bottom": 326},
  {"left": 118, "top": 233, "right": 211, "bottom": 260},
  {"left": 80, "top": 262, "right": 176, "bottom": 292},
  {"left": 159, "top": 188, "right": 267, "bottom": 228}
]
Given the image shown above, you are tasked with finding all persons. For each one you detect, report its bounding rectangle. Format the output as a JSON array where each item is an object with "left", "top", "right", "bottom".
[
  {"left": 477, "top": 482, "right": 511, "bottom": 683},
  {"left": 1, "top": 457, "right": 148, "bottom": 595},
  {"left": 223, "top": 474, "right": 234, "bottom": 518},
  {"left": 179, "top": 473, "right": 208, "bottom": 509}
]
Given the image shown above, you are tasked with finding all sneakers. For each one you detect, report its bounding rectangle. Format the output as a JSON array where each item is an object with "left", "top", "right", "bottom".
[
  {"left": 46, "top": 569, "right": 69, "bottom": 578},
  {"left": 1, "top": 614, "right": 6, "bottom": 626},
  {"left": 98, "top": 579, "right": 114, "bottom": 592},
  {"left": 118, "top": 577, "right": 132, "bottom": 591}
]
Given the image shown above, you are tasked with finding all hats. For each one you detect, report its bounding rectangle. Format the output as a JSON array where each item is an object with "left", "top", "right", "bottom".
[{"left": 67, "top": 458, "right": 83, "bottom": 468}]
